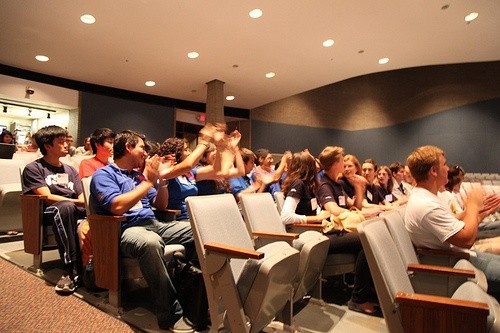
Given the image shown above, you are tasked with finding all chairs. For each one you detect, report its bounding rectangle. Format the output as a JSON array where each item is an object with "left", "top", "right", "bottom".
[{"left": 0, "top": 151, "right": 500, "bottom": 333}]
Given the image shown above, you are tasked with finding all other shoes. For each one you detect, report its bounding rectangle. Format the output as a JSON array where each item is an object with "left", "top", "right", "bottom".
[{"left": 169, "top": 316, "right": 195, "bottom": 333}]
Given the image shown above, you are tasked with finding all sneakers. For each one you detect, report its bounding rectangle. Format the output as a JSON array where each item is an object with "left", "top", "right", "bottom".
[
  {"left": 348, "top": 299, "right": 383, "bottom": 316},
  {"left": 55, "top": 271, "right": 82, "bottom": 292}
]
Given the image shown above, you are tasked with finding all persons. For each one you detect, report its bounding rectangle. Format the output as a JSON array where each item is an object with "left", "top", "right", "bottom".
[
  {"left": 0, "top": 128, "right": 18, "bottom": 160},
  {"left": 80, "top": 127, "right": 322, "bottom": 220},
  {"left": 316, "top": 144, "right": 418, "bottom": 227},
  {"left": 22, "top": 125, "right": 87, "bottom": 294},
  {"left": 281, "top": 149, "right": 380, "bottom": 314},
  {"left": 437, "top": 165, "right": 500, "bottom": 256},
  {"left": 89, "top": 130, "right": 200, "bottom": 333},
  {"left": 407, "top": 145, "right": 500, "bottom": 299},
  {"left": 21, "top": 132, "right": 93, "bottom": 157}
]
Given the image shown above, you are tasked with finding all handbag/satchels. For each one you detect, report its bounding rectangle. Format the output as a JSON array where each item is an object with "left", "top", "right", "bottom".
[
  {"left": 321, "top": 207, "right": 366, "bottom": 234},
  {"left": 178, "top": 262, "right": 209, "bottom": 332},
  {"left": 82, "top": 265, "right": 108, "bottom": 291},
  {"left": 322, "top": 278, "right": 352, "bottom": 305}
]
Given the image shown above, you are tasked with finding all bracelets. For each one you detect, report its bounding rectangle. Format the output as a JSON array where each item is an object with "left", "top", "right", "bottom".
[
  {"left": 156, "top": 180, "right": 169, "bottom": 189},
  {"left": 197, "top": 140, "right": 211, "bottom": 150}
]
